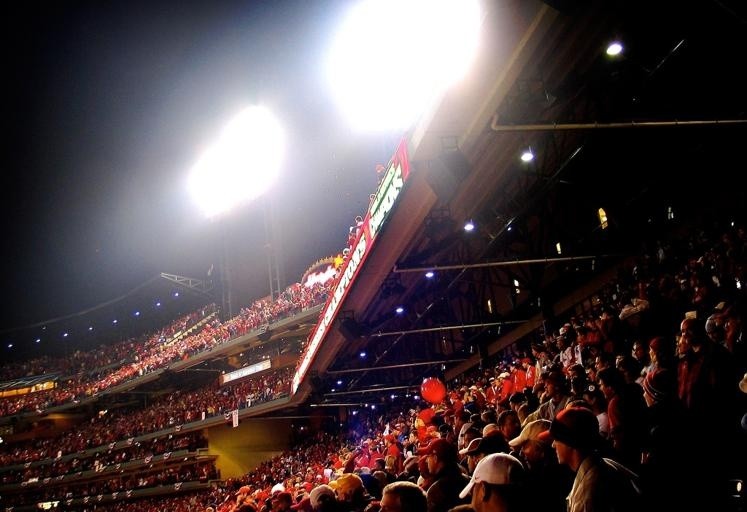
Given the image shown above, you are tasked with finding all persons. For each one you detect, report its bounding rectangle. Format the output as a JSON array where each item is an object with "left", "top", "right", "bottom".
[{"left": 0, "top": 160, "right": 747, "bottom": 510}]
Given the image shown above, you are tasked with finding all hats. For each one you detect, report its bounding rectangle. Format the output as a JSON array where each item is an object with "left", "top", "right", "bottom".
[{"left": 234, "top": 356, "right": 681, "bottom": 512}]
